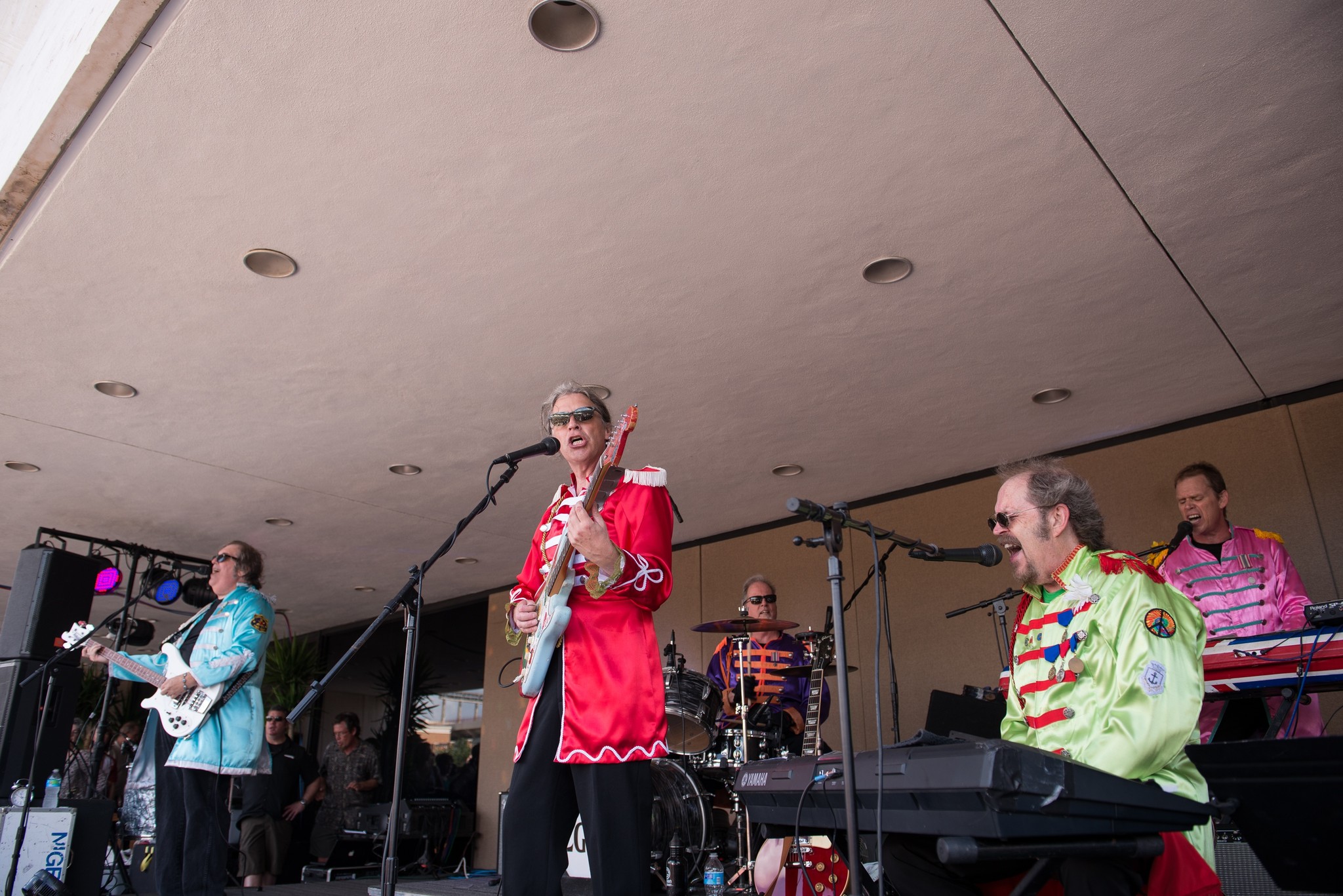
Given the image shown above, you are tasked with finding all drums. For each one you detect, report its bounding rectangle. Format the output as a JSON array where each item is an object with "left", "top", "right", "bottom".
[
  {"left": 658, "top": 666, "right": 719, "bottom": 755},
  {"left": 688, "top": 727, "right": 779, "bottom": 785},
  {"left": 649, "top": 757, "right": 719, "bottom": 887}
]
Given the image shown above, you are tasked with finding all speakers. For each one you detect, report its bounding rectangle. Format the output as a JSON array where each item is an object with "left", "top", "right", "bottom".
[{"left": 0, "top": 546, "right": 112, "bottom": 896}]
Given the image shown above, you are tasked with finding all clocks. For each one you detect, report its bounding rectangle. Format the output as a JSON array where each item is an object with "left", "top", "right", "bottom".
[{"left": 10, "top": 779, "right": 34, "bottom": 808}]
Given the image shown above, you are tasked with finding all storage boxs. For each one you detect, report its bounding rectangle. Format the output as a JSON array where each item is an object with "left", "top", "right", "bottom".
[{"left": 0, "top": 807, "right": 77, "bottom": 896}]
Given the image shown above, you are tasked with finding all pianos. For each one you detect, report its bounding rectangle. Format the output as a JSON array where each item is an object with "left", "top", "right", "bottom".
[
  {"left": 1203, "top": 619, "right": 1343, "bottom": 742},
  {"left": 729, "top": 744, "right": 1225, "bottom": 896}
]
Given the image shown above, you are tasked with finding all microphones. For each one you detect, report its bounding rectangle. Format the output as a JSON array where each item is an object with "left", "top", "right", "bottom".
[
  {"left": 910, "top": 543, "right": 1003, "bottom": 566},
  {"left": 666, "top": 630, "right": 675, "bottom": 668},
  {"left": 492, "top": 436, "right": 561, "bottom": 467},
  {"left": 163, "top": 561, "right": 211, "bottom": 576},
  {"left": 1166, "top": 521, "right": 1193, "bottom": 556}
]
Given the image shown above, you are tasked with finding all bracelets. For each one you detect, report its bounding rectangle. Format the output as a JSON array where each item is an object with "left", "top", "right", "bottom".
[{"left": 183, "top": 673, "right": 192, "bottom": 692}]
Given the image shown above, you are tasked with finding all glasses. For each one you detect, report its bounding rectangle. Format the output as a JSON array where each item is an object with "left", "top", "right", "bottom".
[
  {"left": 987, "top": 506, "right": 1046, "bottom": 532},
  {"left": 266, "top": 715, "right": 284, "bottom": 721},
  {"left": 212, "top": 553, "right": 241, "bottom": 563},
  {"left": 742, "top": 594, "right": 777, "bottom": 605},
  {"left": 547, "top": 406, "right": 604, "bottom": 425}
]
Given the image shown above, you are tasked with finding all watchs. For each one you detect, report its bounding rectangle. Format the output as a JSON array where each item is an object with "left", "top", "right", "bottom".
[{"left": 300, "top": 799, "right": 308, "bottom": 808}]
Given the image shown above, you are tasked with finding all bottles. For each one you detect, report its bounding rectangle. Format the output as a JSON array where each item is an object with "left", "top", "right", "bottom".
[
  {"left": 42, "top": 769, "right": 62, "bottom": 808},
  {"left": 704, "top": 853, "right": 725, "bottom": 896},
  {"left": 666, "top": 832, "right": 689, "bottom": 896}
]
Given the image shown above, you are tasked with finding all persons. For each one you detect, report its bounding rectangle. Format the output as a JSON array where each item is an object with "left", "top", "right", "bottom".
[
  {"left": 704, "top": 574, "right": 831, "bottom": 735},
  {"left": 502, "top": 380, "right": 674, "bottom": 896},
  {"left": 877, "top": 466, "right": 1216, "bottom": 896},
  {"left": 64, "top": 707, "right": 381, "bottom": 887},
  {"left": 407, "top": 732, "right": 481, "bottom": 798},
  {"left": 80, "top": 540, "right": 276, "bottom": 896},
  {"left": 1159, "top": 462, "right": 1325, "bottom": 745}
]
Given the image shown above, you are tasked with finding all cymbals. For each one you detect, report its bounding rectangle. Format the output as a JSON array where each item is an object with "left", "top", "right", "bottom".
[
  {"left": 769, "top": 664, "right": 859, "bottom": 679},
  {"left": 690, "top": 616, "right": 801, "bottom": 634}
]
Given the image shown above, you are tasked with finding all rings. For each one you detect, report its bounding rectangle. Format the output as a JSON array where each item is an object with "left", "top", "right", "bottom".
[{"left": 86, "top": 653, "right": 90, "bottom": 656}]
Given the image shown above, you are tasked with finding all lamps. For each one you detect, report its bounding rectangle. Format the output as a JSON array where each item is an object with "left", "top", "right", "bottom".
[
  {"left": 182, "top": 573, "right": 217, "bottom": 609},
  {"left": 140, "top": 558, "right": 183, "bottom": 604},
  {"left": 106, "top": 615, "right": 155, "bottom": 647},
  {"left": 87, "top": 549, "right": 123, "bottom": 596}
]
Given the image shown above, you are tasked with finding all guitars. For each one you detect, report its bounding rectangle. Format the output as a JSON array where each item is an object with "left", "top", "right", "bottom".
[
  {"left": 56, "top": 619, "right": 224, "bottom": 737},
  {"left": 753, "top": 632, "right": 852, "bottom": 896},
  {"left": 516, "top": 404, "right": 637, "bottom": 700}
]
